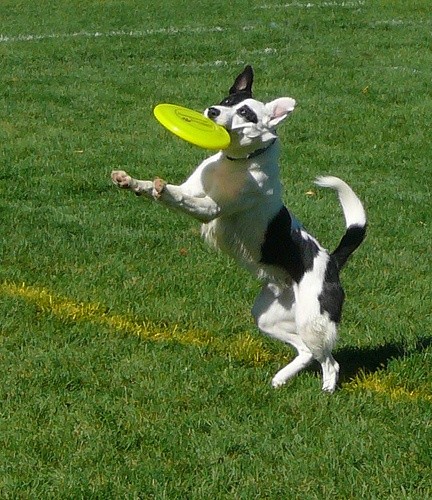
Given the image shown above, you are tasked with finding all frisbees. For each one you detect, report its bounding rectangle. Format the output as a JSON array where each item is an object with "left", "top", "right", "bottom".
[{"left": 153, "top": 103, "right": 231, "bottom": 151}]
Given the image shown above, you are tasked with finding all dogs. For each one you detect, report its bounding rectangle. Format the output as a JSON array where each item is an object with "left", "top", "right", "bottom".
[{"left": 110, "top": 65, "right": 368, "bottom": 395}]
